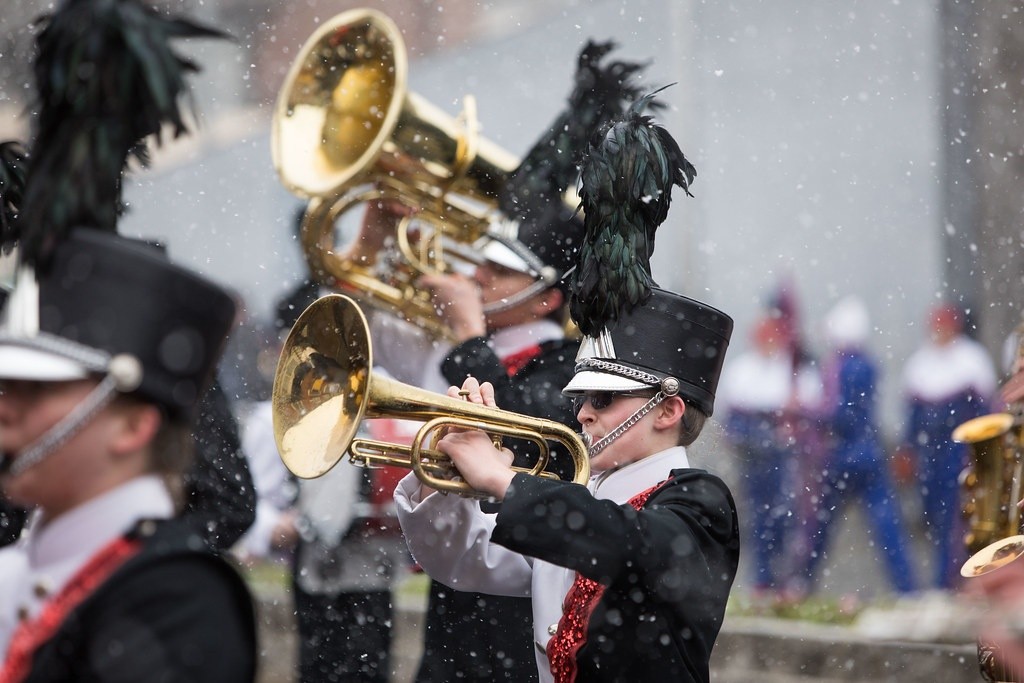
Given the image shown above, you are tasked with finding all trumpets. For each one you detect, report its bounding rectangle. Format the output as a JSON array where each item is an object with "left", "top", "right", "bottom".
[{"left": 271, "top": 293, "right": 594, "bottom": 501}]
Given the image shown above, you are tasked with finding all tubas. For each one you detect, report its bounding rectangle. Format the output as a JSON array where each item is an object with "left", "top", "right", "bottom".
[{"left": 268, "top": 7, "right": 589, "bottom": 345}]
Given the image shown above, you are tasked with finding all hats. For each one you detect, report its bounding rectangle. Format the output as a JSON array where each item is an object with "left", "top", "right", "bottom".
[
  {"left": 561, "top": 81, "right": 733, "bottom": 417},
  {"left": 480, "top": 38, "right": 651, "bottom": 286},
  {"left": 278, "top": 279, "right": 375, "bottom": 335},
  {"left": 0, "top": 0, "right": 235, "bottom": 420}
]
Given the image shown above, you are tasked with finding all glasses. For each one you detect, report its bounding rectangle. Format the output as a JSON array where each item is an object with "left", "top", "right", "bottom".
[{"left": 573, "top": 392, "right": 654, "bottom": 416}]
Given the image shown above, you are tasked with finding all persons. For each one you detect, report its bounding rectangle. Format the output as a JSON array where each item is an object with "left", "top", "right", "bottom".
[
  {"left": 725, "top": 294, "right": 999, "bottom": 599},
  {"left": 269, "top": 200, "right": 741, "bottom": 683},
  {"left": 0, "top": 227, "right": 258, "bottom": 683}
]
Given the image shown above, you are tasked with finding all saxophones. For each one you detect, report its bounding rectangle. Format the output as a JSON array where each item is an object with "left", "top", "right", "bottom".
[{"left": 950, "top": 321, "right": 1024, "bottom": 683}]
